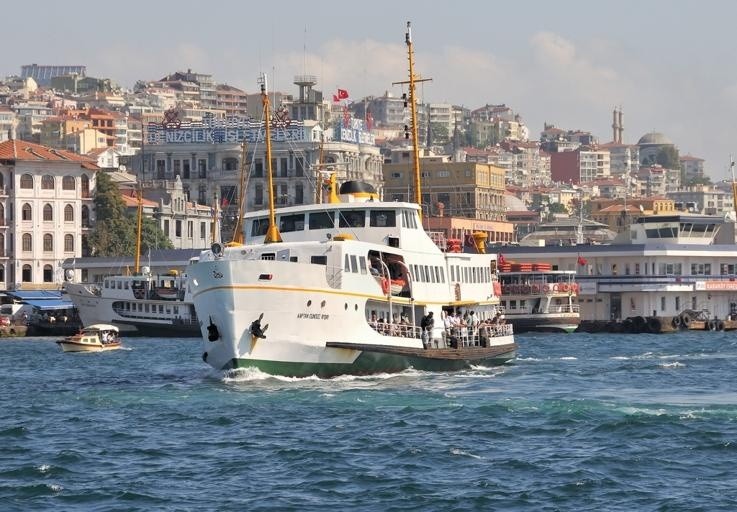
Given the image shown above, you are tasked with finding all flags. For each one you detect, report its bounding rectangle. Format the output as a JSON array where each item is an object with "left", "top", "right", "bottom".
[
  {"left": 331, "top": 95, "right": 339, "bottom": 102},
  {"left": 337, "top": 89, "right": 349, "bottom": 98},
  {"left": 577, "top": 256, "right": 588, "bottom": 265}
]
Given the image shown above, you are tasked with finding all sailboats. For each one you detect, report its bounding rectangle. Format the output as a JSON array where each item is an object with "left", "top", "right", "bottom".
[
  {"left": 180, "top": 70, "right": 517, "bottom": 380},
  {"left": 62, "top": 19, "right": 443, "bottom": 340}
]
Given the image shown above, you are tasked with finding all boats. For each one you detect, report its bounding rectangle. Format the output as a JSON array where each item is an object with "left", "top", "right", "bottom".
[{"left": 57, "top": 324, "right": 125, "bottom": 356}]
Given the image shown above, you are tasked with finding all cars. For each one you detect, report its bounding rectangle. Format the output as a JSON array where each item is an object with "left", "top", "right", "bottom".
[{"left": 0, "top": 304, "right": 73, "bottom": 324}]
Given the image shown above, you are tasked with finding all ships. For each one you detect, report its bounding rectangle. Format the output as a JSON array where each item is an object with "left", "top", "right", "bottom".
[
  {"left": 464, "top": 214, "right": 737, "bottom": 333},
  {"left": 495, "top": 259, "right": 581, "bottom": 333}
]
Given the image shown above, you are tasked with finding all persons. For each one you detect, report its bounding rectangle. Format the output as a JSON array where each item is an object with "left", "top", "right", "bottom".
[
  {"left": 101, "top": 330, "right": 120, "bottom": 344},
  {"left": 366, "top": 309, "right": 506, "bottom": 349},
  {"left": 20, "top": 310, "right": 76, "bottom": 324}
]
